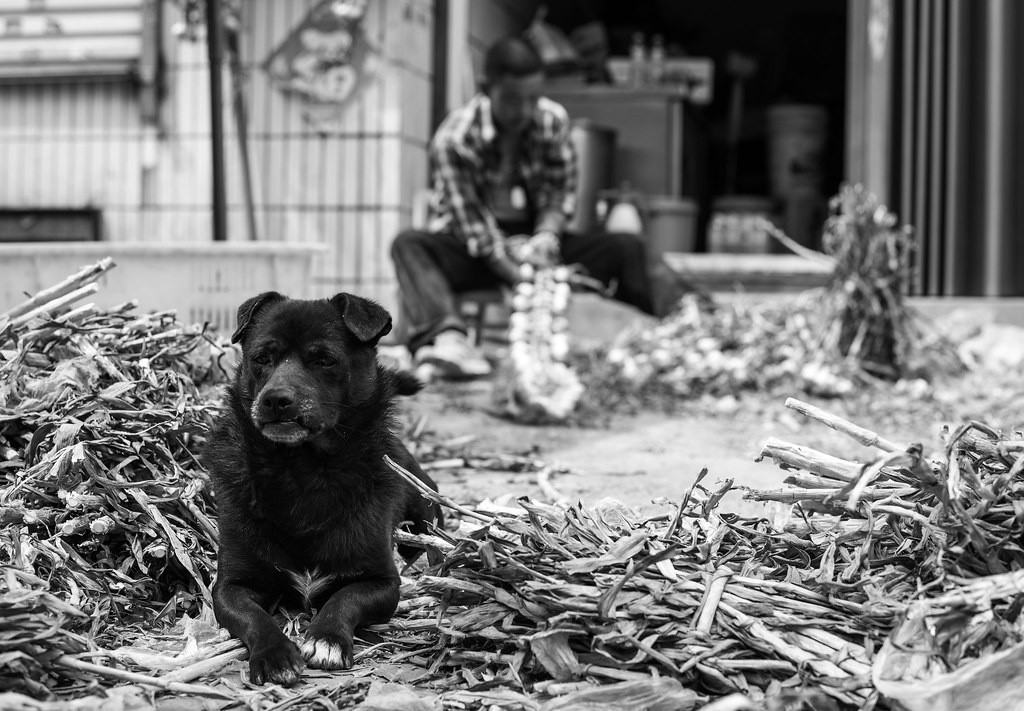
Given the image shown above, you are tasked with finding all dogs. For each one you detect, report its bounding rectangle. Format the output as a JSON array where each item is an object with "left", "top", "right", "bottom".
[{"left": 201, "top": 291, "right": 443, "bottom": 685}]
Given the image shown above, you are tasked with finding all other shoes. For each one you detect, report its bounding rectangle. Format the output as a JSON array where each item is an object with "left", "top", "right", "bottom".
[{"left": 414, "top": 332, "right": 492, "bottom": 383}]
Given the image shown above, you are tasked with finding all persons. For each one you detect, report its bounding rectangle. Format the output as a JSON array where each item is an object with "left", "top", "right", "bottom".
[{"left": 388, "top": 34, "right": 735, "bottom": 381}]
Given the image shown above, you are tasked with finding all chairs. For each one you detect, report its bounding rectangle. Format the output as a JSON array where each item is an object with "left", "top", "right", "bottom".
[{"left": 406, "top": 189, "right": 515, "bottom": 355}]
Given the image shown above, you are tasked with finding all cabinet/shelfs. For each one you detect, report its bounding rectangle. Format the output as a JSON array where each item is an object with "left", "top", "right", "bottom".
[{"left": 495, "top": 84, "right": 714, "bottom": 204}]
